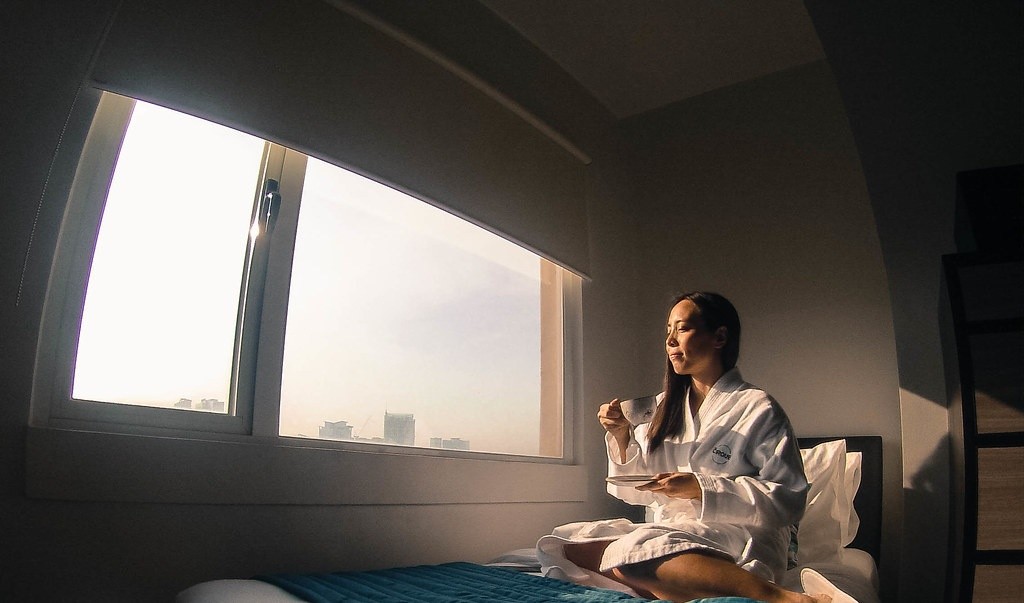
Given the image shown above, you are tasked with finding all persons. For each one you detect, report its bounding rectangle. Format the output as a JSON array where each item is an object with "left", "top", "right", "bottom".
[{"left": 564, "top": 291, "right": 820, "bottom": 603}]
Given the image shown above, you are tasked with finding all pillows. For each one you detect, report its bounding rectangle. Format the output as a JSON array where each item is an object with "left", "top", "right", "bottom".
[
  {"left": 797, "top": 438, "right": 847, "bottom": 562},
  {"left": 845, "top": 452, "right": 862, "bottom": 547}
]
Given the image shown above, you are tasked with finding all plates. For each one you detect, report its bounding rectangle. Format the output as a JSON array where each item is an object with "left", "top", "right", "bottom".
[{"left": 605, "top": 474, "right": 656, "bottom": 485}]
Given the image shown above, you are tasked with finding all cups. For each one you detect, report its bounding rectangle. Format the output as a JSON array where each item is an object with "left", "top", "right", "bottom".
[{"left": 618, "top": 395, "right": 657, "bottom": 429}]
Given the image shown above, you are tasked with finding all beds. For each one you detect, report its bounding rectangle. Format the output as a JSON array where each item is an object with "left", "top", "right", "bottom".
[{"left": 177, "top": 434, "right": 886, "bottom": 603}]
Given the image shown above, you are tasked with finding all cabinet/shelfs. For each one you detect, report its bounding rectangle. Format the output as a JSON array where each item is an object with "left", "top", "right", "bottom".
[{"left": 935, "top": 253, "right": 1024, "bottom": 603}]
